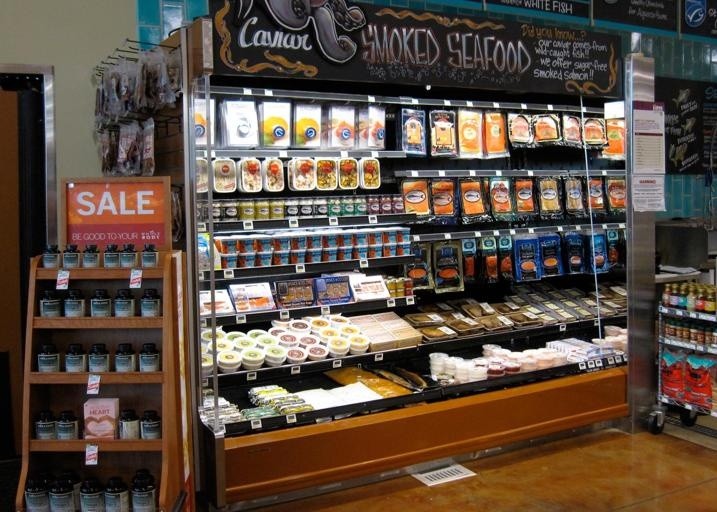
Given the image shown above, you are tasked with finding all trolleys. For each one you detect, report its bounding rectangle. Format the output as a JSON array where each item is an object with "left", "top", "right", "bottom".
[{"left": 649, "top": 301, "right": 717, "bottom": 434}]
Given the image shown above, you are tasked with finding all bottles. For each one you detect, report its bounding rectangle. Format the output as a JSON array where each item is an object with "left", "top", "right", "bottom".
[
  {"left": 663, "top": 282, "right": 717, "bottom": 348},
  {"left": 24, "top": 243, "right": 162, "bottom": 512}
]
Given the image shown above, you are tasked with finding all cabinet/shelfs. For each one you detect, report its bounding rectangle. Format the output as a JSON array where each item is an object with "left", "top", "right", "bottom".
[
  {"left": 91, "top": 10, "right": 632, "bottom": 508},
  {"left": 13, "top": 239, "right": 191, "bottom": 510}
]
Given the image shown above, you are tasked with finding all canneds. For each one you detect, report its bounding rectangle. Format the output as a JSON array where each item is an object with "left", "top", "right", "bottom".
[{"left": 203, "top": 194, "right": 405, "bottom": 223}]
